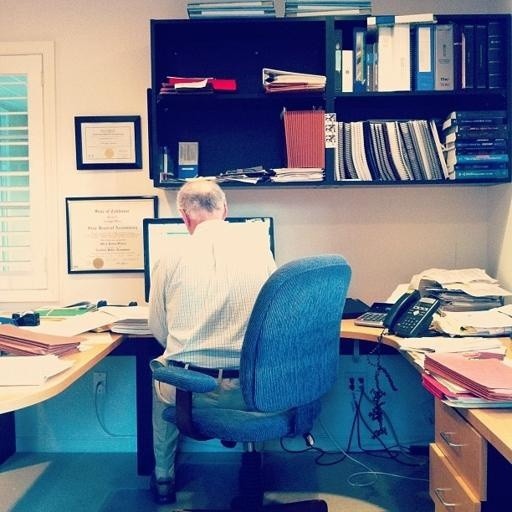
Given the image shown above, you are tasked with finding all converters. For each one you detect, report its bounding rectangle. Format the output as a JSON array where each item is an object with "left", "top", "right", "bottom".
[{"left": 409, "top": 445, "right": 429, "bottom": 455}]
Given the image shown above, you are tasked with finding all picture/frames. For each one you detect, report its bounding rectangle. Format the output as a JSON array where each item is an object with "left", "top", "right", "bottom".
[
  {"left": 66, "top": 196, "right": 157, "bottom": 274},
  {"left": 75, "top": 116, "right": 142, "bottom": 169}
]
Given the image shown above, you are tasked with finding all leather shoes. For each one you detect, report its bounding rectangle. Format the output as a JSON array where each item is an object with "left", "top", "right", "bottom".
[{"left": 148, "top": 471, "right": 175, "bottom": 505}]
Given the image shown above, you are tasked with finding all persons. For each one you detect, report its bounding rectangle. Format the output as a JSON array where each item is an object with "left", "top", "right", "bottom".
[{"left": 146, "top": 178, "right": 283, "bottom": 503}]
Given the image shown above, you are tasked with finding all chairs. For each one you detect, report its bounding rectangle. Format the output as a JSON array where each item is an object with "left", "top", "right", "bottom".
[{"left": 152, "top": 254, "right": 352, "bottom": 512}]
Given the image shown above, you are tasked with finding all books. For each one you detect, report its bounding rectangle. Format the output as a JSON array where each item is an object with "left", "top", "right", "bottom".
[
  {"left": 335, "top": 14, "right": 509, "bottom": 183},
  {"left": 91, "top": 302, "right": 155, "bottom": 337},
  {"left": 160, "top": 72, "right": 238, "bottom": 89},
  {"left": 184, "top": 1, "right": 371, "bottom": 18},
  {"left": 0, "top": 324, "right": 83, "bottom": 352},
  {"left": 388, "top": 267, "right": 511, "bottom": 410},
  {"left": 1, "top": 341, "right": 78, "bottom": 358},
  {"left": 260, "top": 65, "right": 325, "bottom": 92},
  {"left": 269, "top": 166, "right": 328, "bottom": 185}
]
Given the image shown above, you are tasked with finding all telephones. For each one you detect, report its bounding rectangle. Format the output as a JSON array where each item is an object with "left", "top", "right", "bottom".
[{"left": 383, "top": 289, "right": 440, "bottom": 336}]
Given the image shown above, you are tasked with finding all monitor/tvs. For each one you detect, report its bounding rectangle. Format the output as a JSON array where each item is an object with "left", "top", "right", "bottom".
[{"left": 143, "top": 217, "right": 275, "bottom": 304}]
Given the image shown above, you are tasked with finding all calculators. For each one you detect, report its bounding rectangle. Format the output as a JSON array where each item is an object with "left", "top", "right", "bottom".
[{"left": 354, "top": 303, "right": 394, "bottom": 327}]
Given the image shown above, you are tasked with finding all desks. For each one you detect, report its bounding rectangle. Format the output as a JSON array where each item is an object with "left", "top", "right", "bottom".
[{"left": 0, "top": 318, "right": 512, "bottom": 512}]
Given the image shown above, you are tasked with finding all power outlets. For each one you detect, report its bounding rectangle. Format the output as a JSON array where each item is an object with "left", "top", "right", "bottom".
[
  {"left": 344, "top": 373, "right": 366, "bottom": 391},
  {"left": 92, "top": 372, "right": 107, "bottom": 394}
]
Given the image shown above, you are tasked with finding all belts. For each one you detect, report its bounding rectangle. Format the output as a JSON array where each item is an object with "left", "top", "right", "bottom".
[{"left": 169, "top": 361, "right": 239, "bottom": 379}]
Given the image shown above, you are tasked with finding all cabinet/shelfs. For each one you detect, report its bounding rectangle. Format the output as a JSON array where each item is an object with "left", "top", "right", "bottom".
[{"left": 151, "top": 14, "right": 512, "bottom": 188}]
